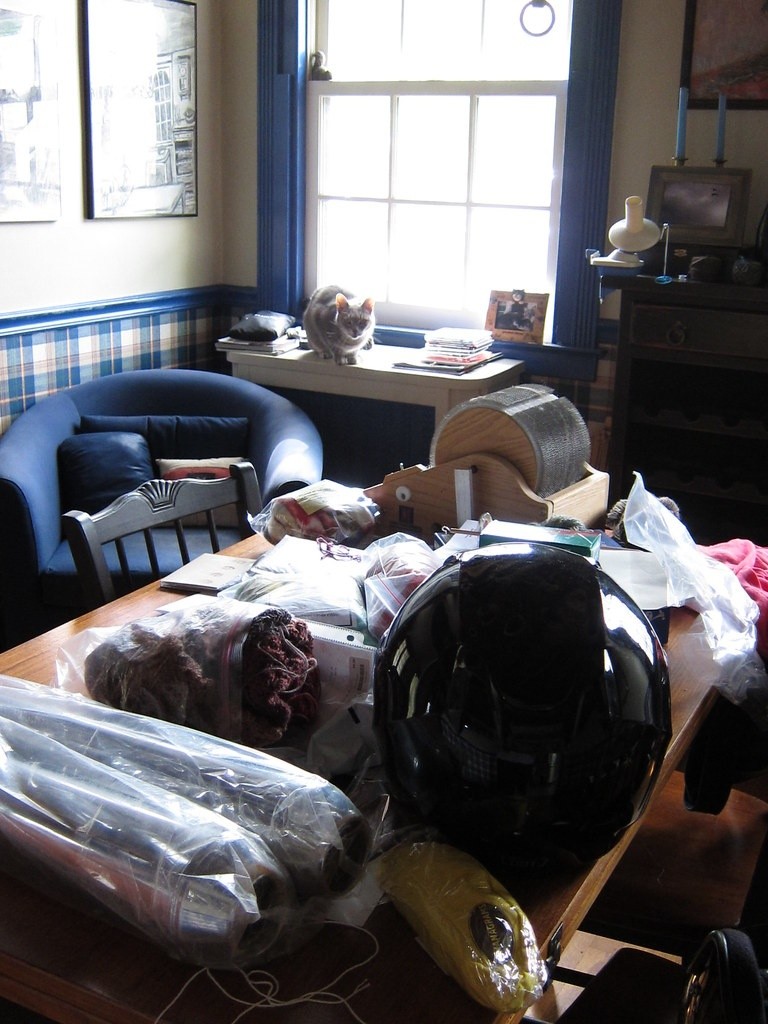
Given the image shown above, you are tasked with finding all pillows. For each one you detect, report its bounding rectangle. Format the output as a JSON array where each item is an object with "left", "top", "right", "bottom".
[
  {"left": 58, "top": 431, "right": 154, "bottom": 538},
  {"left": 154, "top": 457, "right": 249, "bottom": 529},
  {"left": 80, "top": 413, "right": 248, "bottom": 479}
]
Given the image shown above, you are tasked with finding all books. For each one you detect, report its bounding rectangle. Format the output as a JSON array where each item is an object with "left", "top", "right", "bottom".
[
  {"left": 159, "top": 550, "right": 260, "bottom": 593},
  {"left": 214, "top": 332, "right": 302, "bottom": 356},
  {"left": 391, "top": 326, "right": 504, "bottom": 377}
]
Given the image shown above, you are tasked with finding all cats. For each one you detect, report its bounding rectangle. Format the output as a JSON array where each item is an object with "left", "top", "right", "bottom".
[{"left": 302, "top": 285, "right": 375, "bottom": 366}]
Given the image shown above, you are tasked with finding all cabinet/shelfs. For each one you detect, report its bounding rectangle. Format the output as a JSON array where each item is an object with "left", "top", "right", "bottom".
[{"left": 602, "top": 275, "right": 768, "bottom": 547}]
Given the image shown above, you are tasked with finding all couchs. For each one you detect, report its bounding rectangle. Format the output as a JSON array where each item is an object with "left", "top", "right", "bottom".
[{"left": 0, "top": 369, "right": 324, "bottom": 654}]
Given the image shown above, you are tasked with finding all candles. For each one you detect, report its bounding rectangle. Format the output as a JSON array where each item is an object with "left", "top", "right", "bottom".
[
  {"left": 675, "top": 87, "right": 689, "bottom": 159},
  {"left": 715, "top": 93, "right": 727, "bottom": 160}
]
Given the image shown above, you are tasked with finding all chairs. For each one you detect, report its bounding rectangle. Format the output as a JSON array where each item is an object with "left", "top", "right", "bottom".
[
  {"left": 555, "top": 928, "right": 767, "bottom": 1024},
  {"left": 61, "top": 462, "right": 264, "bottom": 611}
]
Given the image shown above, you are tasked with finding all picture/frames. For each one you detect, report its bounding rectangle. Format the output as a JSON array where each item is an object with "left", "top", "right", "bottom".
[
  {"left": 77, "top": 0, "right": 199, "bottom": 220},
  {"left": 676, "top": 0, "right": 768, "bottom": 110},
  {"left": 646, "top": 164, "right": 754, "bottom": 248},
  {"left": 484, "top": 290, "right": 550, "bottom": 345}
]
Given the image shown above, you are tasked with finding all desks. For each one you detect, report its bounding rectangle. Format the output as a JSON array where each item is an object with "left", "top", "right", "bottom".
[
  {"left": 0, "top": 534, "right": 725, "bottom": 1024},
  {"left": 227, "top": 342, "right": 525, "bottom": 431}
]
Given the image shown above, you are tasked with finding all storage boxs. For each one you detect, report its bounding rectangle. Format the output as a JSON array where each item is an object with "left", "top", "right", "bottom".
[
  {"left": 479, "top": 520, "right": 602, "bottom": 559},
  {"left": 593, "top": 528, "right": 670, "bottom": 645}
]
